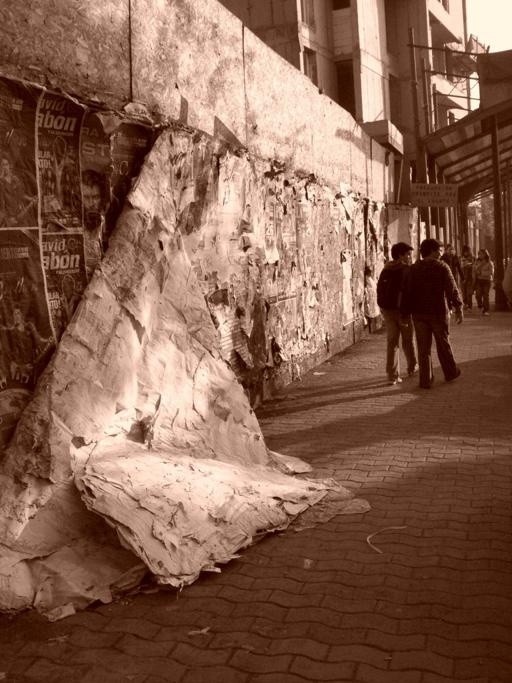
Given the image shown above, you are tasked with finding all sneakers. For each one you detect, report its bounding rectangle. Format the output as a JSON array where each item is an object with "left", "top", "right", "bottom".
[
  {"left": 463, "top": 305, "right": 490, "bottom": 316},
  {"left": 388, "top": 364, "right": 459, "bottom": 388}
]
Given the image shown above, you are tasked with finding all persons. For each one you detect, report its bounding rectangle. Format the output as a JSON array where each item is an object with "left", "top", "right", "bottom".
[
  {"left": 54, "top": 273, "right": 85, "bottom": 335},
  {"left": 0, "top": 151, "right": 40, "bottom": 229},
  {"left": 473, "top": 247, "right": 494, "bottom": 315},
  {"left": 6, "top": 303, "right": 57, "bottom": 394},
  {"left": 79, "top": 168, "right": 110, "bottom": 281},
  {"left": 399, "top": 238, "right": 465, "bottom": 389},
  {"left": 376, "top": 241, "right": 419, "bottom": 385},
  {"left": 458, "top": 240, "right": 477, "bottom": 313},
  {"left": 440, "top": 241, "right": 466, "bottom": 315}
]
